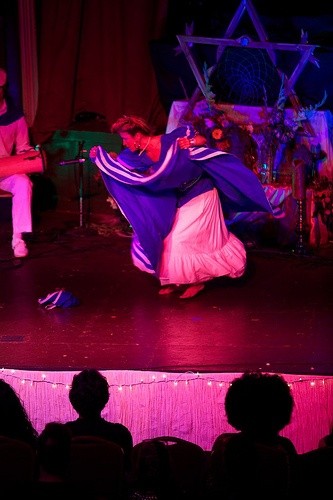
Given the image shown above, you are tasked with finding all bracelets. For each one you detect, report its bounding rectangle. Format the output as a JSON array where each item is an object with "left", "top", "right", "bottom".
[{"left": 189, "top": 138, "right": 196, "bottom": 147}]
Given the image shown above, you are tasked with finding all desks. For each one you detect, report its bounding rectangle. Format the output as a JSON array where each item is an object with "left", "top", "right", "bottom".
[
  {"left": 42, "top": 129, "right": 122, "bottom": 238},
  {"left": 166, "top": 100, "right": 333, "bottom": 184}
]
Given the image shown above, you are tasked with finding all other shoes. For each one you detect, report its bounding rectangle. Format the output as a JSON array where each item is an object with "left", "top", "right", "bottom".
[{"left": 11, "top": 237, "right": 28, "bottom": 257}]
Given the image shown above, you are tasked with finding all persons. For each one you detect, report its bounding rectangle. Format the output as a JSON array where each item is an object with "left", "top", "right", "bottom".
[
  {"left": 89, "top": 114, "right": 274, "bottom": 299},
  {"left": 0, "top": 370, "right": 333, "bottom": 500},
  {"left": 0, "top": 70, "right": 34, "bottom": 258},
  {"left": 183, "top": 106, "right": 333, "bottom": 258}
]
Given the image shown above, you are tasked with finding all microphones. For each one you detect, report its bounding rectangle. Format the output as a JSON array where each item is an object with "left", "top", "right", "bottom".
[{"left": 58, "top": 159, "right": 86, "bottom": 166}]
